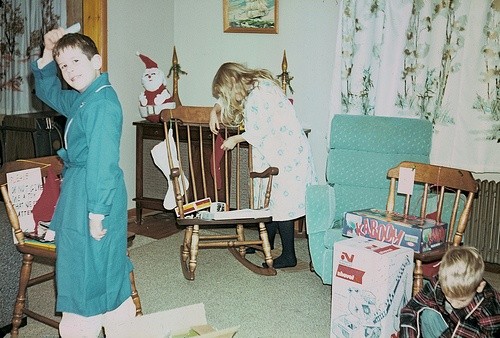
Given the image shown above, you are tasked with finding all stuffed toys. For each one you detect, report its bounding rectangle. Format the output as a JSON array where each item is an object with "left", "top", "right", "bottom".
[{"left": 139, "top": 52, "right": 176, "bottom": 122}]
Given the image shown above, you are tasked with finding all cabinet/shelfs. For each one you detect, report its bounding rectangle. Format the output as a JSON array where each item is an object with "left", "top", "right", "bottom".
[{"left": 132, "top": 119, "right": 253, "bottom": 226}]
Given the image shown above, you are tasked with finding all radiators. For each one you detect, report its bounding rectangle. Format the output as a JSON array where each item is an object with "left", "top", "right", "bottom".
[{"left": 466, "top": 179, "right": 500, "bottom": 266}]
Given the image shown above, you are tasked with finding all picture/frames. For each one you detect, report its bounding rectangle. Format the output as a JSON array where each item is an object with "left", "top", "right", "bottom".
[{"left": 222, "top": 0, "right": 278, "bottom": 34}]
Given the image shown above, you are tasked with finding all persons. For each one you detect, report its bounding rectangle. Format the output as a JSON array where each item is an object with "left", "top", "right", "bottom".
[
  {"left": 399, "top": 247, "right": 500, "bottom": 338},
  {"left": 208, "top": 62, "right": 319, "bottom": 268},
  {"left": 29, "top": 27, "right": 138, "bottom": 338}
]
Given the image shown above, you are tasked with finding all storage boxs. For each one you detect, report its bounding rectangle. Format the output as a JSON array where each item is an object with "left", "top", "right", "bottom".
[
  {"left": 133, "top": 303, "right": 239, "bottom": 338},
  {"left": 341, "top": 206, "right": 449, "bottom": 254},
  {"left": 330, "top": 234, "right": 414, "bottom": 338}
]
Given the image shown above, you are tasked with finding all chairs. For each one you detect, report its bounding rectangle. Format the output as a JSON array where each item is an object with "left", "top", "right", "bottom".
[
  {"left": 305, "top": 112, "right": 468, "bottom": 285},
  {"left": 384, "top": 160, "right": 479, "bottom": 295},
  {"left": 160, "top": 104, "right": 280, "bottom": 281},
  {"left": 1, "top": 175, "right": 143, "bottom": 337}
]
